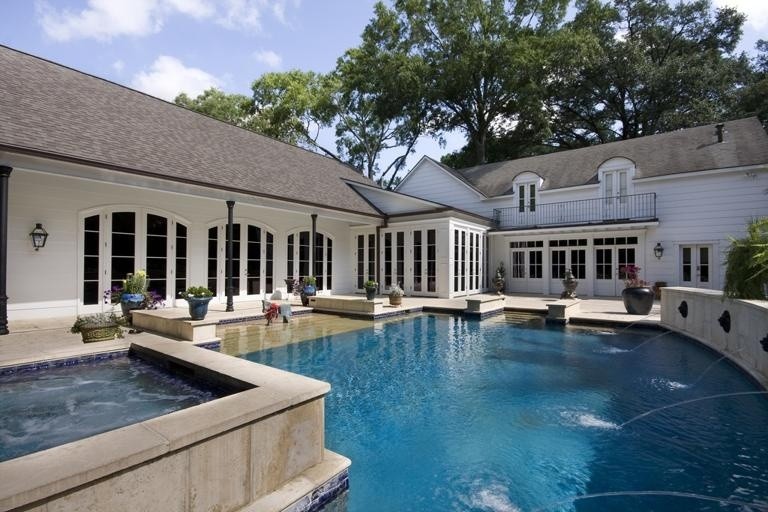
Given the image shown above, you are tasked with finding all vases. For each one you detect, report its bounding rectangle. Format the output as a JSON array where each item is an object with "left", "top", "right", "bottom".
[{"left": 120, "top": 293, "right": 146, "bottom": 324}]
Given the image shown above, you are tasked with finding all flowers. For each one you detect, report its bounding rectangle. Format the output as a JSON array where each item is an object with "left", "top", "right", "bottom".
[
  {"left": 102, "top": 267, "right": 165, "bottom": 309},
  {"left": 621, "top": 266, "right": 650, "bottom": 287}
]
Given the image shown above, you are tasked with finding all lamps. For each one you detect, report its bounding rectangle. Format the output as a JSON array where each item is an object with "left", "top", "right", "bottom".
[
  {"left": 29, "top": 223, "right": 48, "bottom": 251},
  {"left": 654, "top": 242, "right": 663, "bottom": 260}
]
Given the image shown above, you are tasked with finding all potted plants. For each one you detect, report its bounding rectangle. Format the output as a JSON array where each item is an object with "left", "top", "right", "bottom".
[
  {"left": 294, "top": 275, "right": 317, "bottom": 306},
  {"left": 183, "top": 286, "right": 214, "bottom": 320},
  {"left": 389, "top": 282, "right": 404, "bottom": 305},
  {"left": 622, "top": 287, "right": 654, "bottom": 315},
  {"left": 71, "top": 311, "right": 124, "bottom": 342},
  {"left": 363, "top": 281, "right": 379, "bottom": 300}
]
